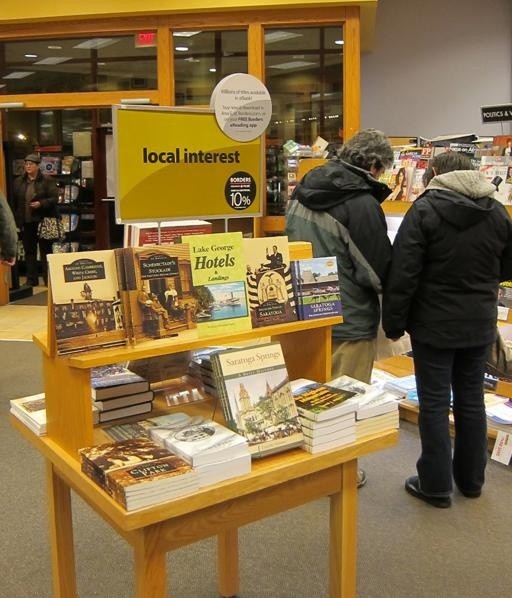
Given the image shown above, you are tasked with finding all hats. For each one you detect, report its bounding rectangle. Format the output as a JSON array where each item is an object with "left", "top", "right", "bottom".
[{"left": 24, "top": 154, "right": 40, "bottom": 163}]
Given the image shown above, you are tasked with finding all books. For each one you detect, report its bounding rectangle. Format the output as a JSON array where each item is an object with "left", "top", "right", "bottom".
[
  {"left": 10, "top": 152, "right": 94, "bottom": 262},
  {"left": 48, "top": 218, "right": 342, "bottom": 356},
  {"left": 372, "top": 366, "right": 512, "bottom": 425},
  {"left": 10, "top": 339, "right": 400, "bottom": 512},
  {"left": 384, "top": 134, "right": 511, "bottom": 315}
]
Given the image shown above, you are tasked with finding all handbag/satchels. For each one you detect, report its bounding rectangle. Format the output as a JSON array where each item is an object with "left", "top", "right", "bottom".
[{"left": 37, "top": 217, "right": 66, "bottom": 242}]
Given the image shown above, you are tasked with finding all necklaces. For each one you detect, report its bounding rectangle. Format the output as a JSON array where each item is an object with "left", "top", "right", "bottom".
[{"left": 12, "top": 152, "right": 61, "bottom": 285}]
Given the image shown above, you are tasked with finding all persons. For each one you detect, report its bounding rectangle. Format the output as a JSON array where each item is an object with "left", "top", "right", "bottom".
[
  {"left": 379, "top": 151, "right": 511, "bottom": 509},
  {"left": 285, "top": 128, "right": 398, "bottom": 492},
  {"left": 0, "top": 194, "right": 19, "bottom": 266}
]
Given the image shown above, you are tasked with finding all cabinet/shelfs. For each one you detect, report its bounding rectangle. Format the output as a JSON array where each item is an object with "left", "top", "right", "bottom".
[
  {"left": 11, "top": 129, "right": 109, "bottom": 272},
  {"left": 296, "top": 143, "right": 512, "bottom": 462},
  {"left": 10, "top": 241, "right": 399, "bottom": 596}
]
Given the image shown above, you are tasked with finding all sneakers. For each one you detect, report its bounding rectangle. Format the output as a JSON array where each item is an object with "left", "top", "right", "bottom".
[{"left": 405, "top": 476, "right": 451, "bottom": 508}]
